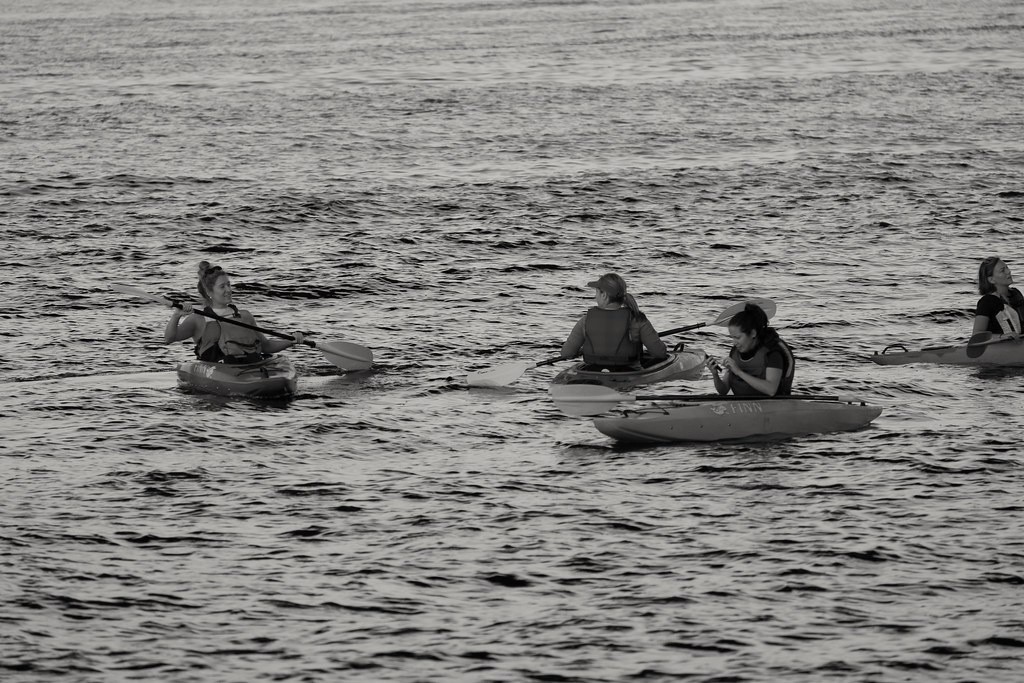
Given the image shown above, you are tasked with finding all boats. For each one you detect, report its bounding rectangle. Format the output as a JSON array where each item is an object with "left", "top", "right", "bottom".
[
  {"left": 594, "top": 396, "right": 883, "bottom": 442},
  {"left": 548, "top": 348, "right": 707, "bottom": 391},
  {"left": 178, "top": 353, "right": 297, "bottom": 398},
  {"left": 870, "top": 333, "right": 1024, "bottom": 368}
]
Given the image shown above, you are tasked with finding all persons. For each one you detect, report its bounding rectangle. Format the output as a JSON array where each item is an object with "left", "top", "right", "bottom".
[
  {"left": 972, "top": 257, "right": 1024, "bottom": 341},
  {"left": 562, "top": 273, "right": 667, "bottom": 373},
  {"left": 165, "top": 261, "right": 303, "bottom": 364},
  {"left": 706, "top": 303, "right": 795, "bottom": 397}
]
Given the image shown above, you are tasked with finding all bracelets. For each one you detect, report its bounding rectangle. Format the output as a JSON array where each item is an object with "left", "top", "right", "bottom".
[{"left": 1000, "top": 334, "right": 1002, "bottom": 338}]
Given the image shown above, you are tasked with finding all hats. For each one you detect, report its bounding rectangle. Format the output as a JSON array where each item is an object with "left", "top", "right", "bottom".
[{"left": 587, "top": 276, "right": 620, "bottom": 299}]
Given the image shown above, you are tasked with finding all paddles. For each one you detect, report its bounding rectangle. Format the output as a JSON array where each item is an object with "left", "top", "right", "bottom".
[
  {"left": 965, "top": 328, "right": 1024, "bottom": 358},
  {"left": 551, "top": 384, "right": 923, "bottom": 416},
  {"left": 111, "top": 284, "right": 373, "bottom": 371},
  {"left": 466, "top": 297, "right": 777, "bottom": 386}
]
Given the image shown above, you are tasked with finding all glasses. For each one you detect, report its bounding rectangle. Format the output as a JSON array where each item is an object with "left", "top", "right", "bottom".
[{"left": 202, "top": 266, "right": 223, "bottom": 282}]
